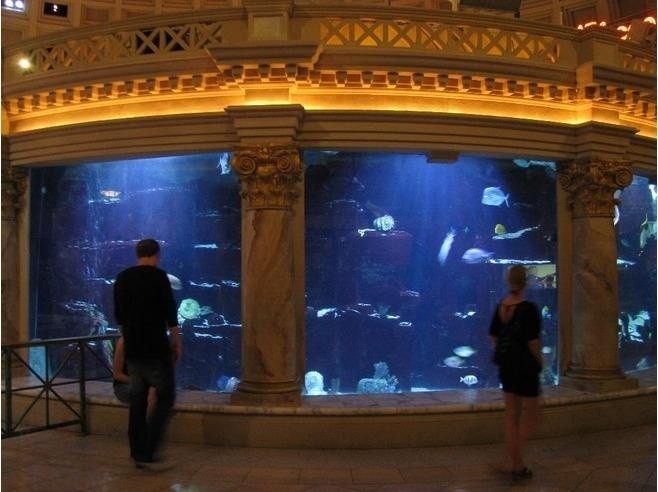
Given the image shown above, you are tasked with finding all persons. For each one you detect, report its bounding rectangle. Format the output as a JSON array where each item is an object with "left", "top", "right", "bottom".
[
  {"left": 110, "top": 333, "right": 158, "bottom": 423},
  {"left": 485, "top": 264, "right": 546, "bottom": 484},
  {"left": 110, "top": 237, "right": 185, "bottom": 476}
]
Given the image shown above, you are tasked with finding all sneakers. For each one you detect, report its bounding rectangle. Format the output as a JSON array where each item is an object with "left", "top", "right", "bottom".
[{"left": 133, "top": 457, "right": 180, "bottom": 474}]
